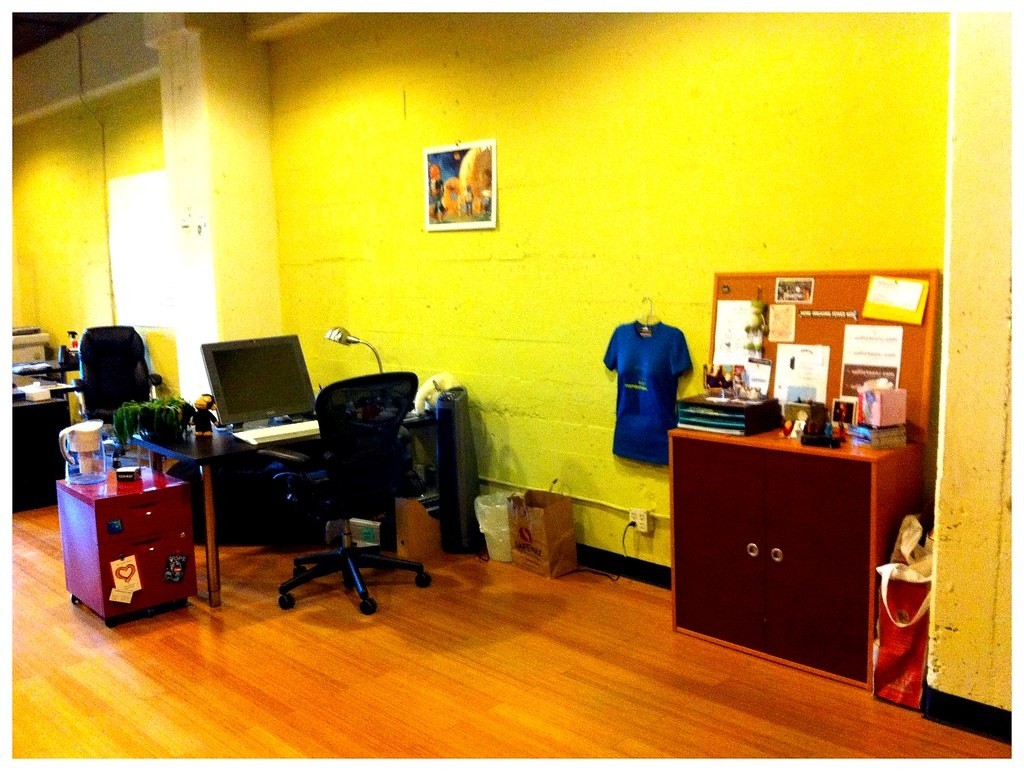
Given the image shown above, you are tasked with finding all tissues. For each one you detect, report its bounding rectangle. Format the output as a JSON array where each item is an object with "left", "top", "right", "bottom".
[
  {"left": 23, "top": 382, "right": 52, "bottom": 402},
  {"left": 860, "top": 377, "right": 908, "bottom": 430}
]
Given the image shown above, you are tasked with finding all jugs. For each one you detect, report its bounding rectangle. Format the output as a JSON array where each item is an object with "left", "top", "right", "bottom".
[{"left": 59, "top": 419, "right": 107, "bottom": 484}]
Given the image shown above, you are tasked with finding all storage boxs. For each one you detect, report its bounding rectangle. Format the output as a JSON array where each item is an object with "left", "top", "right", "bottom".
[{"left": 858, "top": 390, "right": 907, "bottom": 427}]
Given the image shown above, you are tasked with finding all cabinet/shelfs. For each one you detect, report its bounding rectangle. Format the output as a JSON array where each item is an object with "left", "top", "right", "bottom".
[
  {"left": 56, "top": 468, "right": 198, "bottom": 629},
  {"left": 666, "top": 427, "right": 922, "bottom": 693}
]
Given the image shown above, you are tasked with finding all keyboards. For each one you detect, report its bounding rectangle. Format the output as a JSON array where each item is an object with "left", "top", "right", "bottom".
[{"left": 233, "top": 420, "right": 320, "bottom": 446}]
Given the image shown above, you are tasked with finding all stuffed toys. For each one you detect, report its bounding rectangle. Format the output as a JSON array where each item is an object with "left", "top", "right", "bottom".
[{"left": 189, "top": 394, "right": 219, "bottom": 436}]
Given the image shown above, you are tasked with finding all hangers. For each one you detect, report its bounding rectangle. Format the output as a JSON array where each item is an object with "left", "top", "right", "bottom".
[{"left": 635, "top": 297, "right": 661, "bottom": 328}]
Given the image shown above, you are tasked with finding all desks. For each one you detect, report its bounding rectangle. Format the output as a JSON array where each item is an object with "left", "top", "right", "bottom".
[
  {"left": 12, "top": 361, "right": 79, "bottom": 514},
  {"left": 128, "top": 415, "right": 439, "bottom": 607}
]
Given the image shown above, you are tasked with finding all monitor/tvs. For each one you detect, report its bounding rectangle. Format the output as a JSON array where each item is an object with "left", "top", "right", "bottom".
[{"left": 200, "top": 335, "right": 316, "bottom": 431}]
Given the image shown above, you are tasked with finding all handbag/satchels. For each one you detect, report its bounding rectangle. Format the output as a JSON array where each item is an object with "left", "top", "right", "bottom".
[
  {"left": 875, "top": 514, "right": 935, "bottom": 709},
  {"left": 506, "top": 478, "right": 579, "bottom": 580}
]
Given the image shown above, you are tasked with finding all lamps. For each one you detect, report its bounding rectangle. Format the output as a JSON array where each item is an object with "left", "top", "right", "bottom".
[{"left": 326, "top": 328, "right": 383, "bottom": 374}]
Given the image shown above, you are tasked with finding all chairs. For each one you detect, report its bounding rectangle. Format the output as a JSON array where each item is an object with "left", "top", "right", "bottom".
[
  {"left": 72, "top": 325, "right": 163, "bottom": 425},
  {"left": 258, "top": 372, "right": 432, "bottom": 616}
]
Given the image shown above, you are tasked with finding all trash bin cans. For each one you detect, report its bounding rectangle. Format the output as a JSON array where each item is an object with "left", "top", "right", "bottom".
[{"left": 474, "top": 491, "right": 524, "bottom": 563}]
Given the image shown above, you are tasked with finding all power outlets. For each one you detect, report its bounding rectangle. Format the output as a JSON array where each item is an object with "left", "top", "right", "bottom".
[{"left": 628, "top": 508, "right": 655, "bottom": 534}]
